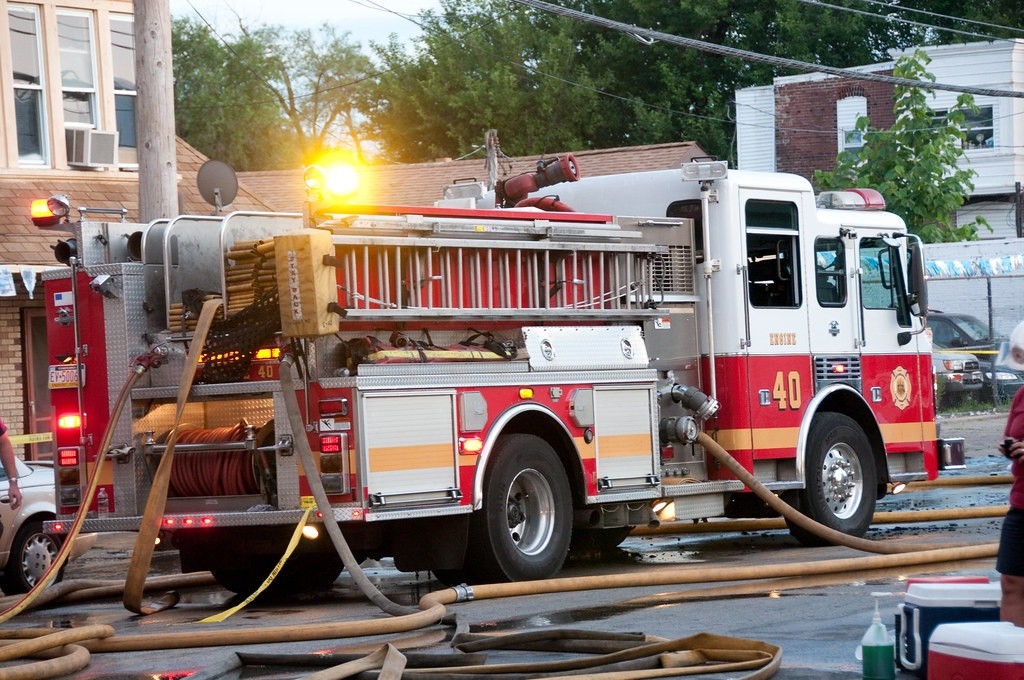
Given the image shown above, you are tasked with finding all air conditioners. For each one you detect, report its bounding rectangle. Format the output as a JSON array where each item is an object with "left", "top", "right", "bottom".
[{"left": 65, "top": 127, "right": 119, "bottom": 167}]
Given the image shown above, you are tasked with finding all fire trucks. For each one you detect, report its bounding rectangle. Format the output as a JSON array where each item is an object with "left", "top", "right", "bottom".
[{"left": 38, "top": 152, "right": 967, "bottom": 596}]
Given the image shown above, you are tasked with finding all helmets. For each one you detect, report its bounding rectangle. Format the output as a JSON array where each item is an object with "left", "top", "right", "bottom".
[{"left": 1007, "top": 321, "right": 1024, "bottom": 371}]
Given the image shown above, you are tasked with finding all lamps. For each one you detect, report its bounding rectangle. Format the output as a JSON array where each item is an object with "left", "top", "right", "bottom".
[{"left": 47, "top": 194, "right": 70, "bottom": 223}]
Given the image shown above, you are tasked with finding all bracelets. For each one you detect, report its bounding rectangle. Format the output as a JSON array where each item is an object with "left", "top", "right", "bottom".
[{"left": 9, "top": 478, "right": 18, "bottom": 482}]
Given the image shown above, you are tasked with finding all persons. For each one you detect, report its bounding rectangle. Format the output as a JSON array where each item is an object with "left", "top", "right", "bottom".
[
  {"left": 995, "top": 321, "right": 1024, "bottom": 628},
  {"left": 0, "top": 418, "right": 23, "bottom": 537}
]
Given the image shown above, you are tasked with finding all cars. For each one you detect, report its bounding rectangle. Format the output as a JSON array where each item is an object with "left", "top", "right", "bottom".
[
  {"left": 0, "top": 450, "right": 98, "bottom": 593},
  {"left": 932, "top": 341, "right": 983, "bottom": 409}
]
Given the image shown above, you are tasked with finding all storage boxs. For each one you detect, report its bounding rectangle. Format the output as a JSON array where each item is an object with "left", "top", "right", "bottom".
[{"left": 895, "top": 576, "right": 1024, "bottom": 680}]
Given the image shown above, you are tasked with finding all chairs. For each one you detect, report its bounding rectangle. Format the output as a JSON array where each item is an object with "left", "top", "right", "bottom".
[{"left": 817, "top": 265, "right": 837, "bottom": 302}]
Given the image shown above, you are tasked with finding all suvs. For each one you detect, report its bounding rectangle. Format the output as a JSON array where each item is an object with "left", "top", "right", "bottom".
[{"left": 926, "top": 308, "right": 1024, "bottom": 405}]
